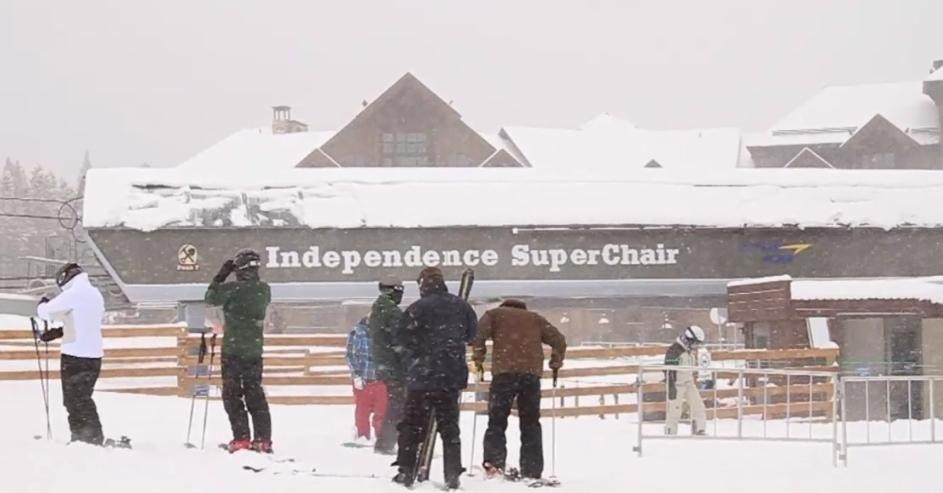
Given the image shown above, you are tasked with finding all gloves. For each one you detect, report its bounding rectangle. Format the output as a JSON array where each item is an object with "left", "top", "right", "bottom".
[
  {"left": 212, "top": 258, "right": 236, "bottom": 282},
  {"left": 36, "top": 295, "right": 49, "bottom": 305},
  {"left": 38, "top": 325, "right": 62, "bottom": 341},
  {"left": 352, "top": 376, "right": 367, "bottom": 390},
  {"left": 668, "top": 383, "right": 677, "bottom": 400}
]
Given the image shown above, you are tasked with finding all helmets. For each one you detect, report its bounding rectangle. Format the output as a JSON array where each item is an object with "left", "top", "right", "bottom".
[
  {"left": 683, "top": 324, "right": 706, "bottom": 343},
  {"left": 230, "top": 246, "right": 262, "bottom": 271}
]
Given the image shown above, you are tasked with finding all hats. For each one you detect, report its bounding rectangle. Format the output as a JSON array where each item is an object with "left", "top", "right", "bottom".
[
  {"left": 414, "top": 265, "right": 446, "bottom": 292},
  {"left": 377, "top": 274, "right": 404, "bottom": 294},
  {"left": 54, "top": 262, "right": 85, "bottom": 287}
]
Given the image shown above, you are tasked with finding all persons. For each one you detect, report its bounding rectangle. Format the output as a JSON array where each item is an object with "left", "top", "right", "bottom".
[
  {"left": 392, "top": 265, "right": 479, "bottom": 490},
  {"left": 342, "top": 314, "right": 386, "bottom": 444},
  {"left": 470, "top": 297, "right": 566, "bottom": 479},
  {"left": 34, "top": 263, "right": 108, "bottom": 446},
  {"left": 662, "top": 325, "right": 709, "bottom": 438},
  {"left": 202, "top": 248, "right": 273, "bottom": 458},
  {"left": 364, "top": 274, "right": 405, "bottom": 451}
]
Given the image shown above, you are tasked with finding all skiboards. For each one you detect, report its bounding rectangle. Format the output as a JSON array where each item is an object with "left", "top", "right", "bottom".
[
  {"left": 413, "top": 268, "right": 475, "bottom": 482},
  {"left": 464, "top": 465, "right": 562, "bottom": 486},
  {"left": 184, "top": 443, "right": 294, "bottom": 463},
  {"left": 33, "top": 435, "right": 132, "bottom": 449},
  {"left": 341, "top": 443, "right": 398, "bottom": 454}
]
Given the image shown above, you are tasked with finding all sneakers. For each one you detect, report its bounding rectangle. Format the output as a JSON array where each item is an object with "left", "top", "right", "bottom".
[
  {"left": 390, "top": 465, "right": 465, "bottom": 488},
  {"left": 483, "top": 465, "right": 543, "bottom": 480},
  {"left": 223, "top": 437, "right": 273, "bottom": 453},
  {"left": 373, "top": 443, "right": 399, "bottom": 456}
]
